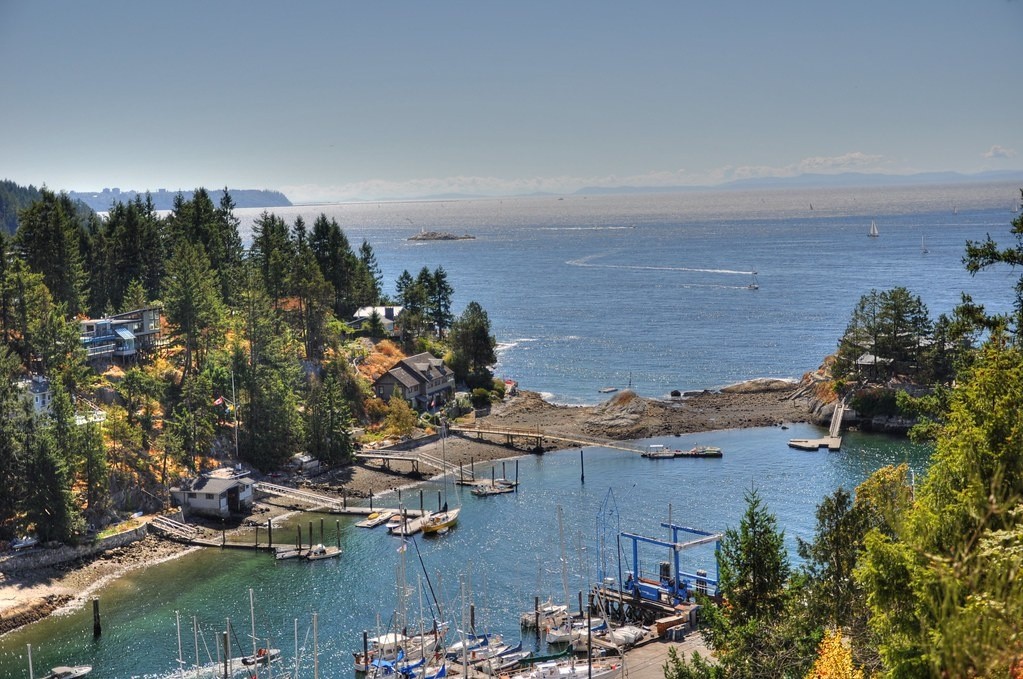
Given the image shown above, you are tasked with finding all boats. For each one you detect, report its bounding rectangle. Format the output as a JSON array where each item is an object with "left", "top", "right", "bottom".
[
  {"left": 643, "top": 443, "right": 675, "bottom": 459},
  {"left": 38, "top": 665, "right": 93, "bottom": 679},
  {"left": 672, "top": 443, "right": 723, "bottom": 458},
  {"left": 244, "top": 644, "right": 281, "bottom": 665},
  {"left": 391, "top": 511, "right": 435, "bottom": 536},
  {"left": 307, "top": 544, "right": 345, "bottom": 560},
  {"left": 355, "top": 508, "right": 395, "bottom": 531},
  {"left": 386, "top": 514, "right": 411, "bottom": 531},
  {"left": 419, "top": 502, "right": 463, "bottom": 533}
]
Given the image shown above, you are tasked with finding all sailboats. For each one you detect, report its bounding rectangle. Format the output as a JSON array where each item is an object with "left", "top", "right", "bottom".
[
  {"left": 865, "top": 217, "right": 879, "bottom": 236},
  {"left": 747, "top": 269, "right": 760, "bottom": 290},
  {"left": 453, "top": 492, "right": 706, "bottom": 679},
  {"left": 354, "top": 501, "right": 501, "bottom": 679}
]
